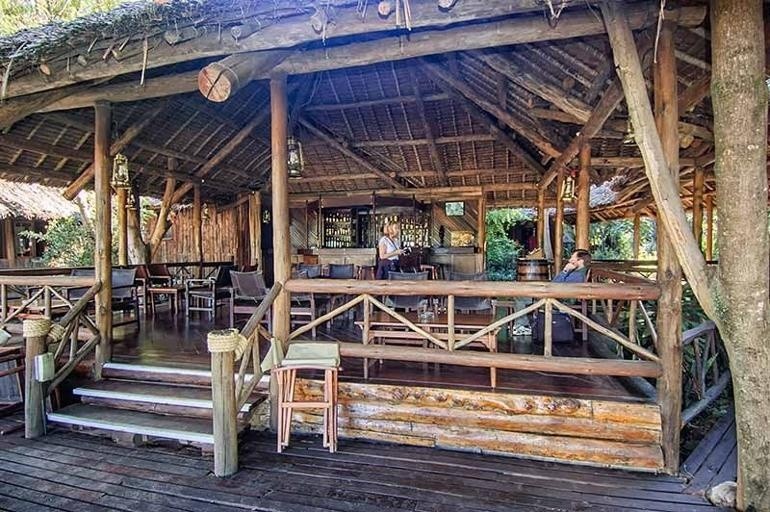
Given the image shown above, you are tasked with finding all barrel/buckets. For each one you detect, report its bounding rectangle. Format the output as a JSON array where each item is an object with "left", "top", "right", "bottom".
[{"left": 515, "top": 257, "right": 550, "bottom": 281}]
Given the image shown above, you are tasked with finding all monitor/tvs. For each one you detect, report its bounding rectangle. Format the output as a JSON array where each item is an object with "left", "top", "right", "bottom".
[{"left": 445, "top": 200, "right": 465, "bottom": 217}]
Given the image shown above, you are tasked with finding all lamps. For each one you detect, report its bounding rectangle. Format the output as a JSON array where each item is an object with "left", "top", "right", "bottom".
[
  {"left": 111, "top": 151, "right": 131, "bottom": 189},
  {"left": 286, "top": 135, "right": 306, "bottom": 182}
]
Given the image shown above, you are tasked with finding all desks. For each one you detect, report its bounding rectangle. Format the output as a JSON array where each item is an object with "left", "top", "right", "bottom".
[
  {"left": 491, "top": 300, "right": 517, "bottom": 331},
  {"left": 6, "top": 297, "right": 91, "bottom": 340},
  {"left": 352, "top": 310, "right": 499, "bottom": 387}
]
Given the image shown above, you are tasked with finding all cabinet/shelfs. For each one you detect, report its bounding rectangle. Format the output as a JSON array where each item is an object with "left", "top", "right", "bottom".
[{"left": 319, "top": 206, "right": 430, "bottom": 264}]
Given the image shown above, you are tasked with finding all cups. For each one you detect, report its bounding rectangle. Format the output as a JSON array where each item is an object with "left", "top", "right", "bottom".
[{"left": 416, "top": 302, "right": 427, "bottom": 318}]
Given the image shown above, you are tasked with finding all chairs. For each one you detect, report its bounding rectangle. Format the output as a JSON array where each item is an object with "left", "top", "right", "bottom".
[
  {"left": 288, "top": 265, "right": 362, "bottom": 338},
  {"left": 367, "top": 265, "right": 495, "bottom": 336},
  {"left": 539, "top": 268, "right": 591, "bottom": 341},
  {"left": 69, "top": 266, "right": 141, "bottom": 331},
  {"left": 136, "top": 264, "right": 273, "bottom": 334}
]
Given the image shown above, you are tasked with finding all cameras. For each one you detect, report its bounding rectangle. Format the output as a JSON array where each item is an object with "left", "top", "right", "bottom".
[{"left": 401, "top": 246, "right": 412, "bottom": 256}]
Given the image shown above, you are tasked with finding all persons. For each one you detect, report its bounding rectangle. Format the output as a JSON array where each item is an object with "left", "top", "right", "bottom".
[
  {"left": 508, "top": 249, "right": 591, "bottom": 336},
  {"left": 376, "top": 220, "right": 406, "bottom": 310}
]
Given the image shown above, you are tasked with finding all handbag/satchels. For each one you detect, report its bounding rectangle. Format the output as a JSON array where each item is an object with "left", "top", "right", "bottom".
[{"left": 537, "top": 311, "right": 574, "bottom": 343}]
